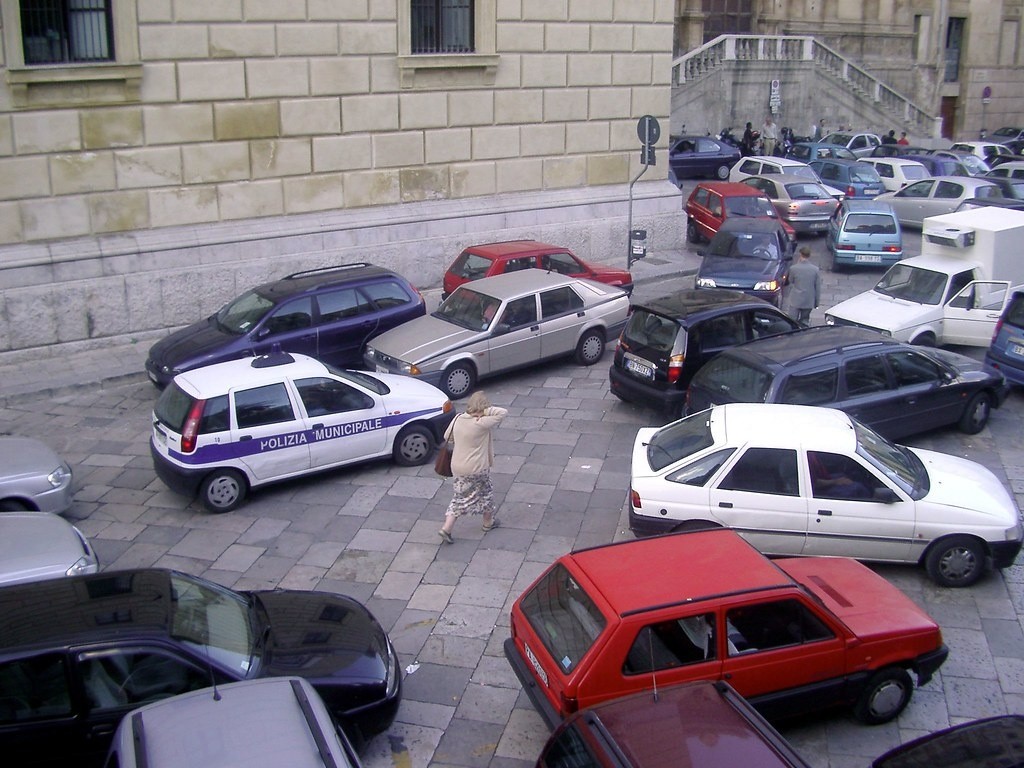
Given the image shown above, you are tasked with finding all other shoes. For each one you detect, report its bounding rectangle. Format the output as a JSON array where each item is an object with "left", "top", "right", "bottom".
[
  {"left": 438, "top": 528, "right": 454, "bottom": 544},
  {"left": 482, "top": 516, "right": 502, "bottom": 531}
]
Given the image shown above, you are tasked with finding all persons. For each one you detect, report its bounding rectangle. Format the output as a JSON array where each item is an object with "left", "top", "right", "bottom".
[
  {"left": 881, "top": 130, "right": 898, "bottom": 155},
  {"left": 787, "top": 244, "right": 821, "bottom": 323},
  {"left": 761, "top": 115, "right": 778, "bottom": 156},
  {"left": 897, "top": 131, "right": 909, "bottom": 154},
  {"left": 438, "top": 390, "right": 507, "bottom": 544},
  {"left": 815, "top": 118, "right": 826, "bottom": 141},
  {"left": 744, "top": 121, "right": 754, "bottom": 150}
]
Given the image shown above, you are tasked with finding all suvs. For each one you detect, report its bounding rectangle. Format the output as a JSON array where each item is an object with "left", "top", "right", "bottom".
[
  {"left": 144, "top": 262, "right": 427, "bottom": 389},
  {"left": 531, "top": 678, "right": 818, "bottom": 767},
  {"left": 0, "top": 568, "right": 405, "bottom": 768},
  {"left": 680, "top": 324, "right": 1010, "bottom": 443}
]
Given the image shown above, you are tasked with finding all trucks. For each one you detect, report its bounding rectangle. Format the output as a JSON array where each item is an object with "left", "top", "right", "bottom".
[{"left": 823, "top": 203, "right": 1024, "bottom": 349}]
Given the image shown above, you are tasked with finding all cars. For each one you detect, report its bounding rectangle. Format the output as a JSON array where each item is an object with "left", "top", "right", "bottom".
[
  {"left": 983, "top": 286, "right": 1024, "bottom": 385},
  {"left": 609, "top": 288, "right": 813, "bottom": 411},
  {"left": 863, "top": 714, "right": 1024, "bottom": 768},
  {"left": 363, "top": 268, "right": 630, "bottom": 401},
  {"left": 693, "top": 218, "right": 797, "bottom": 307},
  {"left": 608, "top": 287, "right": 814, "bottom": 421},
  {"left": 669, "top": 136, "right": 744, "bottom": 182},
  {"left": 728, "top": 127, "right": 1024, "bottom": 274},
  {"left": 503, "top": 525, "right": 950, "bottom": 731},
  {"left": 148, "top": 342, "right": 457, "bottom": 514},
  {"left": 685, "top": 181, "right": 797, "bottom": 260},
  {"left": 437, "top": 239, "right": 634, "bottom": 319},
  {"left": 628, "top": 401, "right": 1023, "bottom": 588},
  {"left": 0, "top": 434, "right": 101, "bottom": 589}
]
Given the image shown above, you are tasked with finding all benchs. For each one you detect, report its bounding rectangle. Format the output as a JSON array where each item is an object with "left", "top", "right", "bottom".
[{"left": 562, "top": 587, "right": 679, "bottom": 676}]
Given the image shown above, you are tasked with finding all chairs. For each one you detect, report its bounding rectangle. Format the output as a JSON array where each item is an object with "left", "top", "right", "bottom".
[
  {"left": 99, "top": 654, "right": 187, "bottom": 695},
  {"left": 83, "top": 659, "right": 129, "bottom": 708}
]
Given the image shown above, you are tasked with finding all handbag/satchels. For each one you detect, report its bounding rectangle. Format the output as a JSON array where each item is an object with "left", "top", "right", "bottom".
[{"left": 434, "top": 412, "right": 461, "bottom": 477}]
